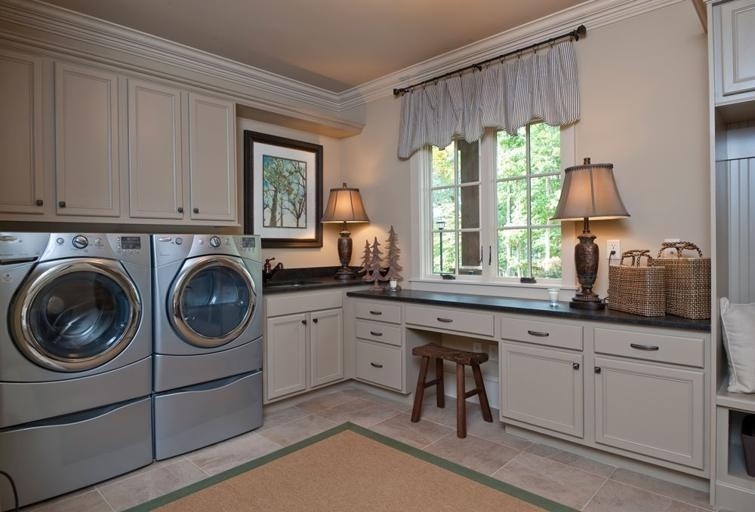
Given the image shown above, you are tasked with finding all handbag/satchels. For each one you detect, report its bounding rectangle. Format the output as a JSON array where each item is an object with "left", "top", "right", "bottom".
[
  {"left": 646, "top": 240, "right": 712, "bottom": 321},
  {"left": 606, "top": 249, "right": 667, "bottom": 319}
]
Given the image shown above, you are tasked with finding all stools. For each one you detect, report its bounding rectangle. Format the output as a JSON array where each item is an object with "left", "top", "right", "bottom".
[{"left": 410, "top": 342, "right": 493, "bottom": 439}]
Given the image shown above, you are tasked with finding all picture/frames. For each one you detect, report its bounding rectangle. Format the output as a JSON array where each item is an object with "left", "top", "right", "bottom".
[{"left": 240, "top": 128, "right": 326, "bottom": 253}]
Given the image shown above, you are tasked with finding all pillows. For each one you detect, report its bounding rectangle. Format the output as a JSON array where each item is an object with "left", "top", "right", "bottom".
[{"left": 719, "top": 295, "right": 755, "bottom": 395}]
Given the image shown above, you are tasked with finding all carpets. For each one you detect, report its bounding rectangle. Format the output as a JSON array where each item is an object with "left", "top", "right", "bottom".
[{"left": 121, "top": 420, "right": 580, "bottom": 512}]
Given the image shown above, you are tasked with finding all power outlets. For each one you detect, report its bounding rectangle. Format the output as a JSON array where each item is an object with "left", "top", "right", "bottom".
[{"left": 606, "top": 239, "right": 621, "bottom": 262}]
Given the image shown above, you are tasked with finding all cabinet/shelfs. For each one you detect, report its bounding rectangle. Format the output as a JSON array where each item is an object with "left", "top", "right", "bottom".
[
  {"left": 692, "top": 0, "right": 755, "bottom": 511},
  {"left": 498, "top": 307, "right": 589, "bottom": 450},
  {"left": 122, "top": 66, "right": 239, "bottom": 229},
  {"left": 403, "top": 300, "right": 498, "bottom": 344},
  {"left": 262, "top": 288, "right": 352, "bottom": 406},
  {"left": 352, "top": 296, "right": 442, "bottom": 396},
  {"left": 0, "top": 38, "right": 125, "bottom": 228},
  {"left": 592, "top": 320, "right": 716, "bottom": 483}
]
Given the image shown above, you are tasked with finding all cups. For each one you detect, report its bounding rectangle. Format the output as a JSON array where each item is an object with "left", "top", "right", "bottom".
[
  {"left": 547, "top": 288, "right": 561, "bottom": 305},
  {"left": 390, "top": 279, "right": 397, "bottom": 292}
]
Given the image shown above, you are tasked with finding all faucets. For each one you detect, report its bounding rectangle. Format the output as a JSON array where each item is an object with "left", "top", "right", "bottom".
[{"left": 262, "top": 258, "right": 284, "bottom": 280}]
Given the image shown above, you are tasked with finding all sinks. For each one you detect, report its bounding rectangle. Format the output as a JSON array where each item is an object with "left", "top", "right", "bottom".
[{"left": 265, "top": 280, "right": 324, "bottom": 290}]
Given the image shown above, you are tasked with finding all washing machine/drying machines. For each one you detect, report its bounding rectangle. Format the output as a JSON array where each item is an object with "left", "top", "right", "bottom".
[
  {"left": 154, "top": 233, "right": 265, "bottom": 462},
  {"left": 0, "top": 231, "right": 152, "bottom": 511}
]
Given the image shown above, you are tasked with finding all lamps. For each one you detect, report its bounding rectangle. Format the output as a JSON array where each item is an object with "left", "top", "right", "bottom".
[
  {"left": 551, "top": 154, "right": 632, "bottom": 313},
  {"left": 320, "top": 179, "right": 370, "bottom": 281}
]
